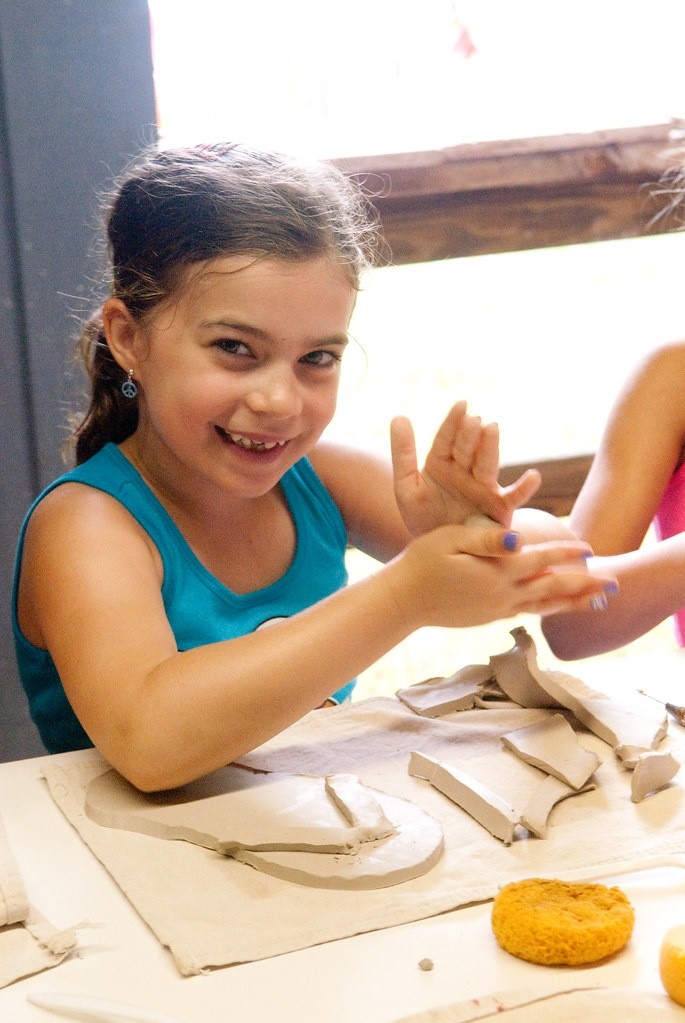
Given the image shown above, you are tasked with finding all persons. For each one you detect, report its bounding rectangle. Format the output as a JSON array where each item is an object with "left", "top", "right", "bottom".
[
  {"left": 543, "top": 337, "right": 685, "bottom": 659},
  {"left": 9, "top": 141, "right": 617, "bottom": 793}
]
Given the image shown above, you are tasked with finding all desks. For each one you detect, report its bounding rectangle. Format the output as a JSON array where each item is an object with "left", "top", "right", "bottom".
[{"left": 0, "top": 670, "right": 685, "bottom": 1023}]
